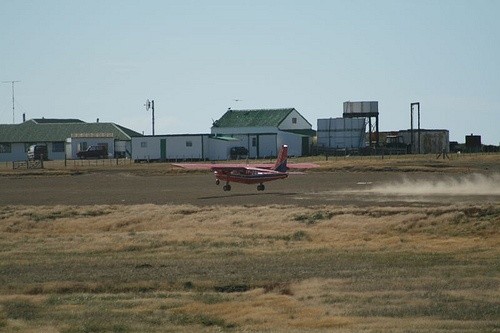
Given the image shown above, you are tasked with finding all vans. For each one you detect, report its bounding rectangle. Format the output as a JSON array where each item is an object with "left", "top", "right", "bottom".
[{"left": 26, "top": 145, "right": 48, "bottom": 160}]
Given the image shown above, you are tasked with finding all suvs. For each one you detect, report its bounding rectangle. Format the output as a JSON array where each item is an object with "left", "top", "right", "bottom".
[{"left": 77, "top": 146, "right": 107, "bottom": 159}]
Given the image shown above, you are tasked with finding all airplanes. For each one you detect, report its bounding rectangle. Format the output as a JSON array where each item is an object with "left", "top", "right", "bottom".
[{"left": 174, "top": 144, "right": 320, "bottom": 191}]
